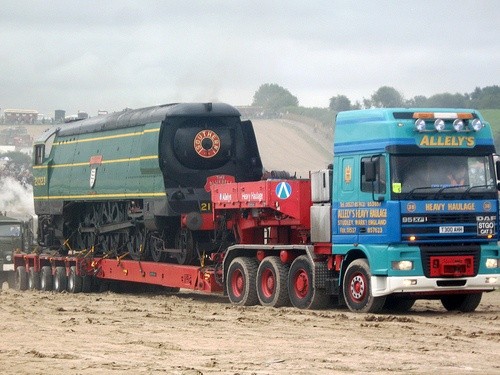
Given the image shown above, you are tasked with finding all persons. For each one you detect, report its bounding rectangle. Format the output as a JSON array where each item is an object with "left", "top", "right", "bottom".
[
  {"left": 446, "top": 158, "right": 470, "bottom": 192},
  {"left": 240, "top": 107, "right": 334, "bottom": 141},
  {"left": 0, "top": 116, "right": 77, "bottom": 192}
]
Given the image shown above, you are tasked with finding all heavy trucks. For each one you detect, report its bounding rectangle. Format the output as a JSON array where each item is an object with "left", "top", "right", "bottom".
[
  {"left": 0, "top": 213, "right": 35, "bottom": 289},
  {"left": 29, "top": 102, "right": 500, "bottom": 313}
]
